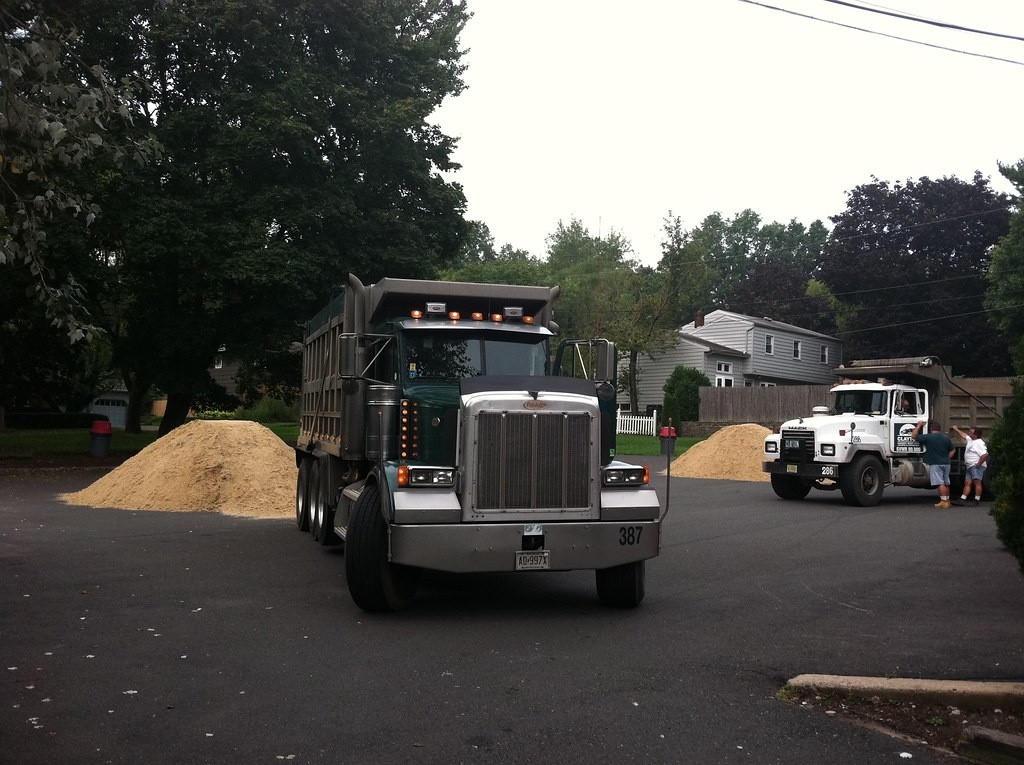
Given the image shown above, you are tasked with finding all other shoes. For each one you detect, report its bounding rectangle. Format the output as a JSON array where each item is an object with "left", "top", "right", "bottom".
[
  {"left": 967, "top": 499, "right": 980, "bottom": 507},
  {"left": 934, "top": 501, "right": 951, "bottom": 508},
  {"left": 950, "top": 497, "right": 966, "bottom": 506}
]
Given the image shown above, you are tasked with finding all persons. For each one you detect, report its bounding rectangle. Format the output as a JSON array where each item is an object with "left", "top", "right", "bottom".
[
  {"left": 951, "top": 425, "right": 989, "bottom": 506},
  {"left": 894, "top": 400, "right": 909, "bottom": 415},
  {"left": 912, "top": 421, "right": 955, "bottom": 507}
]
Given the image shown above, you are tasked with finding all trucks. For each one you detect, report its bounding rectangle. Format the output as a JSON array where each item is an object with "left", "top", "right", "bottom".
[
  {"left": 291, "top": 278, "right": 678, "bottom": 610},
  {"left": 760, "top": 380, "right": 1003, "bottom": 506}
]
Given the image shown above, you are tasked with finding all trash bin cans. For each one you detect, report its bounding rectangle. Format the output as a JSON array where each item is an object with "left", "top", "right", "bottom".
[
  {"left": 659, "top": 426, "right": 677, "bottom": 454},
  {"left": 90, "top": 420, "right": 112, "bottom": 458}
]
